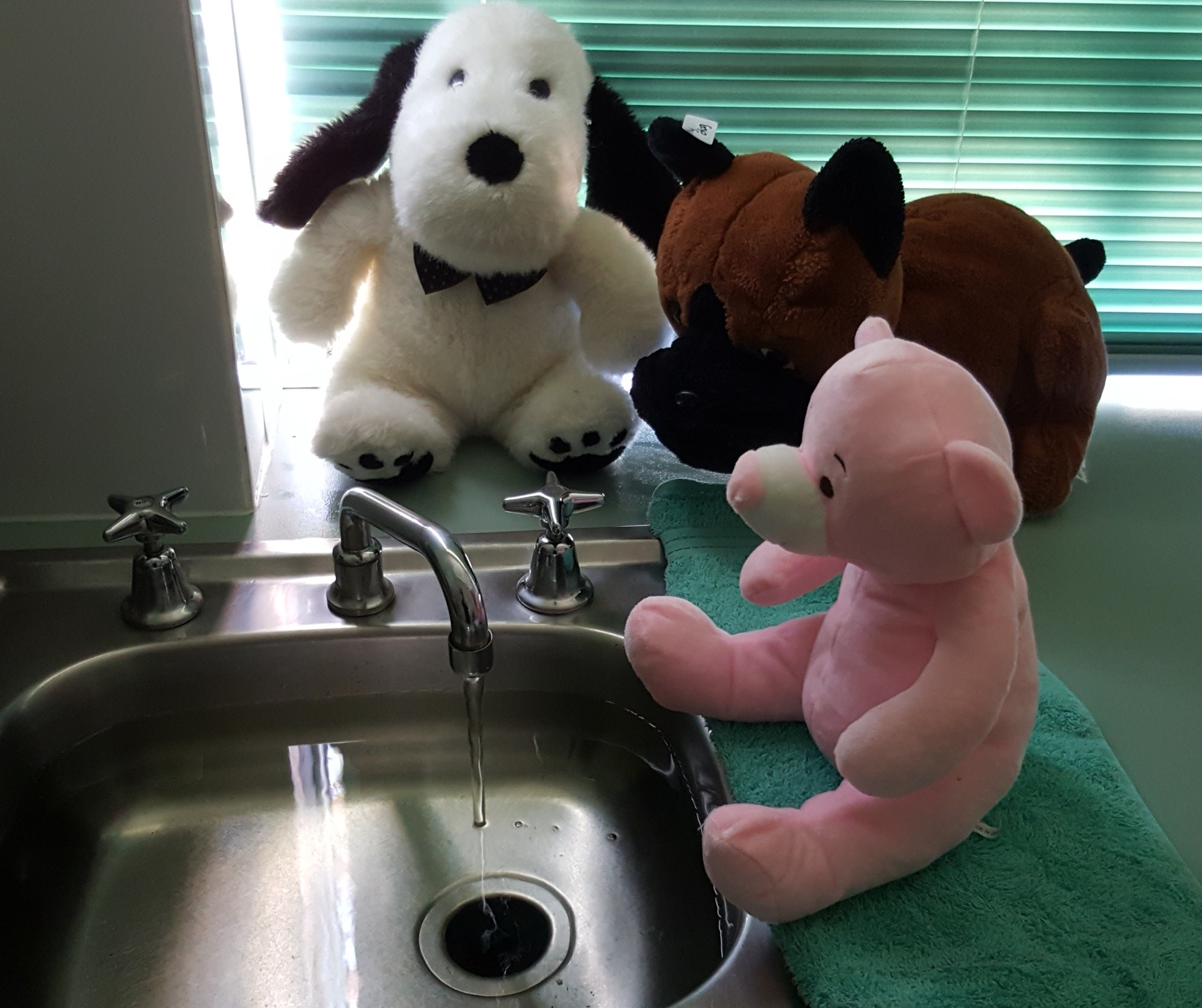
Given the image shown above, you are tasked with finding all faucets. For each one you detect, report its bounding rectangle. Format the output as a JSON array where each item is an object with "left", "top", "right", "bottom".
[{"left": 326, "top": 485, "right": 502, "bottom": 679}]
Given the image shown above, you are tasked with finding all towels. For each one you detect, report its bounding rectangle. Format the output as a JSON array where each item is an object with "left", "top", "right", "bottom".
[{"left": 644, "top": 475, "right": 1201, "bottom": 1008}]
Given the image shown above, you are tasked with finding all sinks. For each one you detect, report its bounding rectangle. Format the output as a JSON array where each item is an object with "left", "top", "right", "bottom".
[{"left": 0, "top": 524, "right": 801, "bottom": 1008}]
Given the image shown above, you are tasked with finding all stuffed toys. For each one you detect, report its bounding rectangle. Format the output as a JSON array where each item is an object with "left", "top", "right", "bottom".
[
  {"left": 628, "top": 312, "right": 1037, "bottom": 922},
  {"left": 252, "top": 2, "right": 686, "bottom": 493},
  {"left": 620, "top": 117, "right": 1107, "bottom": 525}
]
